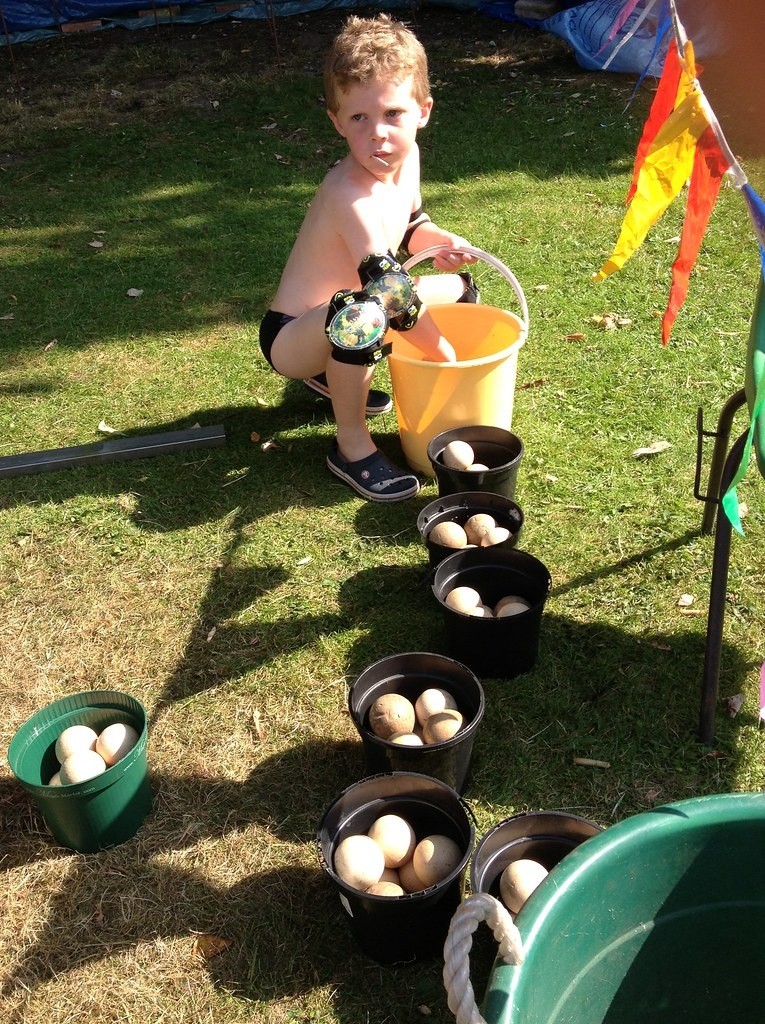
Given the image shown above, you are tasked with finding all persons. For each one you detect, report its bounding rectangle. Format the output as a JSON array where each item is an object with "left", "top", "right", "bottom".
[{"left": 258, "top": 13, "right": 476, "bottom": 504}]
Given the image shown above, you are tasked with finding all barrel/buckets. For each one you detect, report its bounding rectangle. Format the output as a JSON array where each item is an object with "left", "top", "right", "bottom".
[
  {"left": 378, "top": 244, "right": 530, "bottom": 477},
  {"left": 483, "top": 793, "right": 765, "bottom": 1024},
  {"left": 469, "top": 809, "right": 604, "bottom": 922},
  {"left": 430, "top": 546, "right": 553, "bottom": 681},
  {"left": 8, "top": 691, "right": 153, "bottom": 855},
  {"left": 417, "top": 492, "right": 525, "bottom": 583},
  {"left": 318, "top": 770, "right": 475, "bottom": 971},
  {"left": 427, "top": 425, "right": 524, "bottom": 501},
  {"left": 348, "top": 652, "right": 486, "bottom": 796}
]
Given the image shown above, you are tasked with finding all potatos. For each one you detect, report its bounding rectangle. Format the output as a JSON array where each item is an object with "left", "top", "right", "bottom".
[
  {"left": 47, "top": 723, "right": 138, "bottom": 787},
  {"left": 333, "top": 439, "right": 550, "bottom": 927}
]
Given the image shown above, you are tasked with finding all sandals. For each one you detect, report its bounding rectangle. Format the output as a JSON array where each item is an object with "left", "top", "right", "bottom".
[
  {"left": 303, "top": 372, "right": 393, "bottom": 416},
  {"left": 326, "top": 436, "right": 420, "bottom": 502}
]
